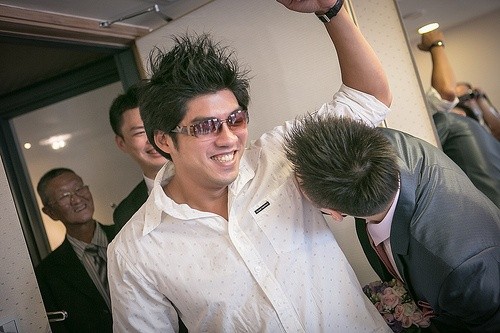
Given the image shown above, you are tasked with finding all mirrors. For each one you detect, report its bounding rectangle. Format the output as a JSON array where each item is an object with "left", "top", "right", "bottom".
[{"left": 394, "top": 0, "right": 499, "bottom": 207}]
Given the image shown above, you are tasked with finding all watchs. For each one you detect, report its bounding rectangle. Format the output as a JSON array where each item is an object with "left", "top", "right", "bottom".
[{"left": 426, "top": 40, "right": 447, "bottom": 50}]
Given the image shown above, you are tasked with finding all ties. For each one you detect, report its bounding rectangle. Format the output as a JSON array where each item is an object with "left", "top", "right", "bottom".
[
  {"left": 366, "top": 226, "right": 404, "bottom": 285},
  {"left": 85, "top": 245, "right": 110, "bottom": 298}
]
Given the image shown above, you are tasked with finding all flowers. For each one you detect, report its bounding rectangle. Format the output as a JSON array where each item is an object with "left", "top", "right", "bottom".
[{"left": 362, "top": 279, "right": 433, "bottom": 333}]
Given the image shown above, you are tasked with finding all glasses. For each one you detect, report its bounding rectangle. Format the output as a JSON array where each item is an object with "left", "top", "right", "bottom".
[
  {"left": 47, "top": 183, "right": 89, "bottom": 205},
  {"left": 172, "top": 107, "right": 250, "bottom": 139}
]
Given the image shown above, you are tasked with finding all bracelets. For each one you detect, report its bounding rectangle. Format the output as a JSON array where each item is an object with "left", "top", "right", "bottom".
[{"left": 316, "top": 0, "right": 345, "bottom": 24}]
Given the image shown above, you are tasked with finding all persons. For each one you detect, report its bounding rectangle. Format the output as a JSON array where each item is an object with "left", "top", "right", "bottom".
[
  {"left": 293, "top": 117, "right": 500, "bottom": 333},
  {"left": 106, "top": 0, "right": 394, "bottom": 333},
  {"left": 417, "top": 28, "right": 500, "bottom": 208},
  {"left": 33, "top": 167, "right": 122, "bottom": 333},
  {"left": 109, "top": 82, "right": 170, "bottom": 230}
]
